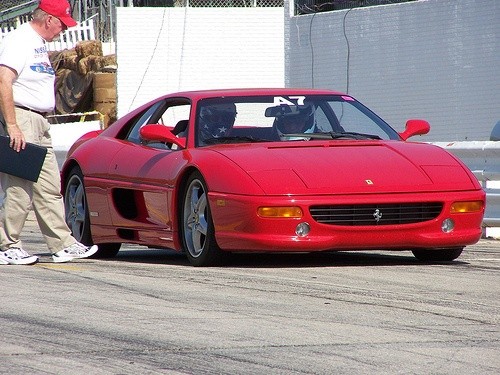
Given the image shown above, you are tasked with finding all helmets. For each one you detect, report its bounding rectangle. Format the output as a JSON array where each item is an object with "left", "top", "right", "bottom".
[
  {"left": 275, "top": 100, "right": 316, "bottom": 141},
  {"left": 200, "top": 102, "right": 236, "bottom": 140}
]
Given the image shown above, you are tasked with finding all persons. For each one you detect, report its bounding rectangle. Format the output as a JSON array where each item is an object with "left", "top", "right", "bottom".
[
  {"left": 0, "top": 0, "right": 101, "bottom": 265},
  {"left": 171, "top": 102, "right": 238, "bottom": 150}
]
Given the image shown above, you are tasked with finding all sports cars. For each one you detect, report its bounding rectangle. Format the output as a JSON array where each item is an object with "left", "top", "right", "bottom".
[{"left": 58, "top": 88, "right": 488, "bottom": 264}]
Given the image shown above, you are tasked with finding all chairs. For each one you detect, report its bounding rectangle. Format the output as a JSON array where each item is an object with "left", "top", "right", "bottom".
[{"left": 165, "top": 120, "right": 189, "bottom": 147}]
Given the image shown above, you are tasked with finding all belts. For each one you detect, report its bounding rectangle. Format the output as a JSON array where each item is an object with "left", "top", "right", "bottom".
[{"left": 14, "top": 105, "right": 48, "bottom": 117}]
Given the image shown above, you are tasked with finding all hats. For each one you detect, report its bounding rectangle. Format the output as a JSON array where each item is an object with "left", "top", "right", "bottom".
[{"left": 38, "top": 0, "right": 78, "bottom": 27}]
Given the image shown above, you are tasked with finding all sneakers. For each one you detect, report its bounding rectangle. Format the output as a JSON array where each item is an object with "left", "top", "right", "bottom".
[
  {"left": 52, "top": 242, "right": 99, "bottom": 262},
  {"left": 0, "top": 248, "right": 39, "bottom": 265}
]
